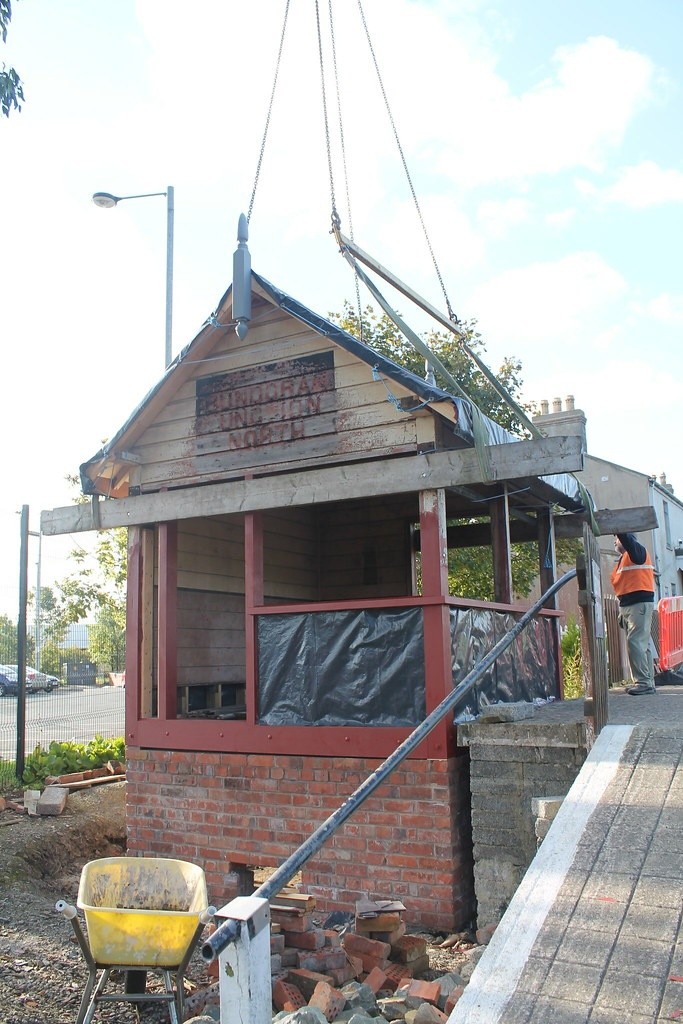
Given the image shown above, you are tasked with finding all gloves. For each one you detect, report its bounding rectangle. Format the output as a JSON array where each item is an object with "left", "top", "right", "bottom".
[{"left": 617, "top": 613, "right": 623, "bottom": 628}]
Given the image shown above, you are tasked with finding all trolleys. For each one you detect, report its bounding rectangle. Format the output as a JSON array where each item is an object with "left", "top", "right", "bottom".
[{"left": 55, "top": 856, "right": 218, "bottom": 1023}]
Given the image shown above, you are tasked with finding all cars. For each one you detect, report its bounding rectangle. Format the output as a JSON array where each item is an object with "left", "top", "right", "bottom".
[
  {"left": 6, "top": 665, "right": 47, "bottom": 695},
  {"left": 44, "top": 674, "right": 59, "bottom": 693},
  {"left": 0, "top": 665, "right": 32, "bottom": 697}
]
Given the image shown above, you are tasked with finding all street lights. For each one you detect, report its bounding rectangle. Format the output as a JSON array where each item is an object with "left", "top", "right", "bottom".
[{"left": 92, "top": 186, "right": 174, "bottom": 370}]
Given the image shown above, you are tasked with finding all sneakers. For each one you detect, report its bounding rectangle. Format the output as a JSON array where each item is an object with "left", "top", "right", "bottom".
[
  {"left": 627, "top": 686, "right": 654, "bottom": 695},
  {"left": 626, "top": 686, "right": 656, "bottom": 691}
]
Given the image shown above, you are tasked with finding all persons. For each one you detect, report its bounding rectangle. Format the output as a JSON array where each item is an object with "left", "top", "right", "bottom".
[{"left": 599, "top": 507, "right": 656, "bottom": 695}]
